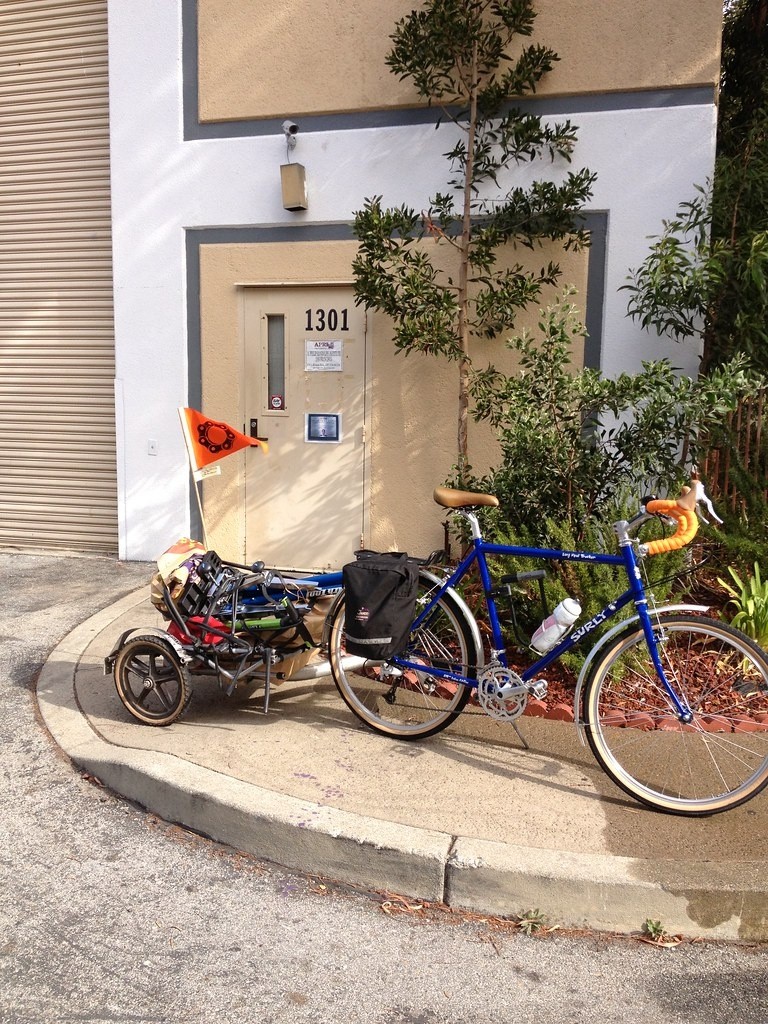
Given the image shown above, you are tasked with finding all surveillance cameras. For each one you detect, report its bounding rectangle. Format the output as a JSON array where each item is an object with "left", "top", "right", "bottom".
[{"left": 282, "top": 120, "right": 299, "bottom": 135}]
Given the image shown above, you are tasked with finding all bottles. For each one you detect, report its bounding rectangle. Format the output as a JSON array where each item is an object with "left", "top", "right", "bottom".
[{"left": 531, "top": 598, "right": 582, "bottom": 653}]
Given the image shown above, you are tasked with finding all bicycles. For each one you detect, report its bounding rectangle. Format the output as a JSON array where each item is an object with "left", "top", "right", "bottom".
[{"left": 321, "top": 465, "right": 768, "bottom": 818}]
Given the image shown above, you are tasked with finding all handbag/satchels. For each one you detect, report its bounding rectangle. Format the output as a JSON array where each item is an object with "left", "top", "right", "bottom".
[{"left": 340, "top": 552, "right": 418, "bottom": 661}]
